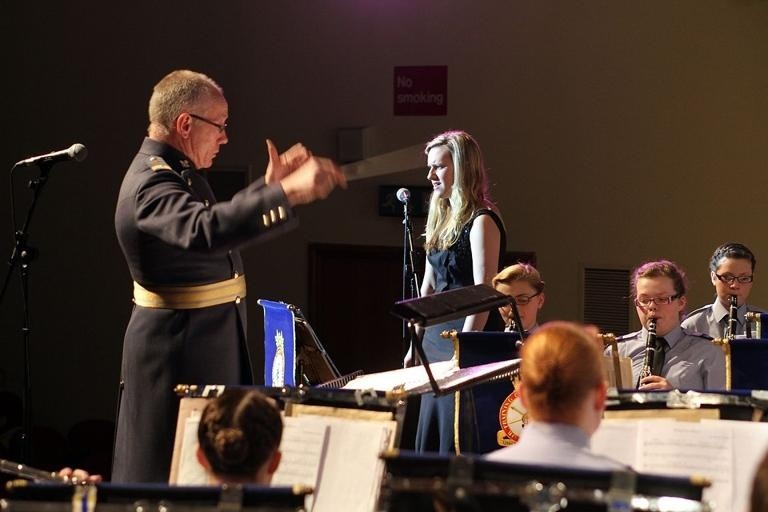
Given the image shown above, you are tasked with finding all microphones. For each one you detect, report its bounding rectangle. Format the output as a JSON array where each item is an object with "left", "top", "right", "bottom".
[
  {"left": 15, "top": 143, "right": 89, "bottom": 168},
  {"left": 396, "top": 187, "right": 412, "bottom": 202}
]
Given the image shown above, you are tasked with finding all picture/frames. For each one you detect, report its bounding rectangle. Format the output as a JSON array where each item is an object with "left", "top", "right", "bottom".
[{"left": 202, "top": 124, "right": 367, "bottom": 203}]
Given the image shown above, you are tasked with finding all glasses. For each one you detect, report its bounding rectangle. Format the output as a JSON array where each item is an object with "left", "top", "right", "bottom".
[
  {"left": 713, "top": 272, "right": 757, "bottom": 286},
  {"left": 513, "top": 290, "right": 538, "bottom": 309},
  {"left": 633, "top": 294, "right": 679, "bottom": 309},
  {"left": 185, "top": 111, "right": 228, "bottom": 133}
]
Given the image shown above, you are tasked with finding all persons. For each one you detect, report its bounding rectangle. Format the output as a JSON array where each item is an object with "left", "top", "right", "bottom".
[
  {"left": 490, "top": 263, "right": 544, "bottom": 336},
  {"left": 403, "top": 132, "right": 505, "bottom": 453},
  {"left": 681, "top": 241, "right": 767, "bottom": 342},
  {"left": 109, "top": 69, "right": 349, "bottom": 486},
  {"left": 480, "top": 320, "right": 635, "bottom": 471},
  {"left": 603, "top": 262, "right": 727, "bottom": 391},
  {"left": 195, "top": 389, "right": 284, "bottom": 486}
]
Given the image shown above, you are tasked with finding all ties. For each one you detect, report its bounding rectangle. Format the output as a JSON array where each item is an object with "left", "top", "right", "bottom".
[{"left": 652, "top": 337, "right": 668, "bottom": 376}]
